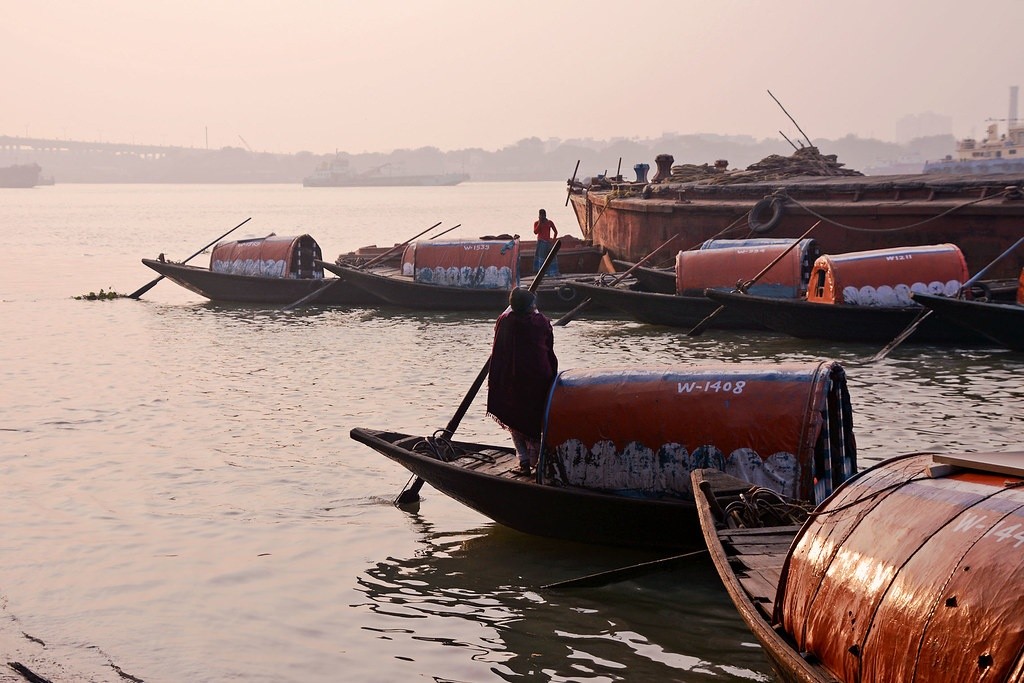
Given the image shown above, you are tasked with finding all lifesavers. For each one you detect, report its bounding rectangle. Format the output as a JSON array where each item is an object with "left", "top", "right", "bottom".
[
  {"left": 747, "top": 196, "right": 783, "bottom": 234},
  {"left": 557, "top": 285, "right": 579, "bottom": 303}
]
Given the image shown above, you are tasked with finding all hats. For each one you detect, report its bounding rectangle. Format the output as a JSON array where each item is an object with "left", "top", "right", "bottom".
[{"left": 509, "top": 286, "right": 533, "bottom": 311}]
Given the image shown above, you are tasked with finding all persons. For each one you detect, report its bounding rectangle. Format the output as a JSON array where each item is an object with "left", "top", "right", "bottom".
[
  {"left": 486, "top": 286, "right": 558, "bottom": 476},
  {"left": 533, "top": 209, "right": 561, "bottom": 276}
]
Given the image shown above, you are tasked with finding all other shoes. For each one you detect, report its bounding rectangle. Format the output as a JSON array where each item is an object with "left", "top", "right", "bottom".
[{"left": 508, "top": 465, "right": 537, "bottom": 477}]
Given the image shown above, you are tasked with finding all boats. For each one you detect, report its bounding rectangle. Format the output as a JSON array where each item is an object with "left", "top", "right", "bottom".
[
  {"left": 690, "top": 451, "right": 1024, "bottom": 683},
  {"left": 565, "top": 244, "right": 803, "bottom": 329},
  {"left": 565, "top": 154, "right": 1024, "bottom": 279},
  {"left": 612, "top": 239, "right": 820, "bottom": 295},
  {"left": 335, "top": 234, "right": 608, "bottom": 274},
  {"left": 313, "top": 239, "right": 638, "bottom": 310},
  {"left": 142, "top": 232, "right": 376, "bottom": 304},
  {"left": 910, "top": 278, "right": 1024, "bottom": 353},
  {"left": 704, "top": 243, "right": 1021, "bottom": 342},
  {"left": 302, "top": 159, "right": 471, "bottom": 187},
  {"left": 350, "top": 360, "right": 856, "bottom": 548}
]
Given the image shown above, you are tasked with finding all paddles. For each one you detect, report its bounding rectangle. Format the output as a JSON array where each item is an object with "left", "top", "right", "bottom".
[
  {"left": 284, "top": 220, "right": 445, "bottom": 309},
  {"left": 687, "top": 218, "right": 821, "bottom": 338},
  {"left": 381, "top": 223, "right": 461, "bottom": 267},
  {"left": 127, "top": 216, "right": 252, "bottom": 297},
  {"left": 542, "top": 547, "right": 714, "bottom": 593},
  {"left": 552, "top": 232, "right": 679, "bottom": 325},
  {"left": 877, "top": 235, "right": 1024, "bottom": 359},
  {"left": 395, "top": 236, "right": 565, "bottom": 511}
]
